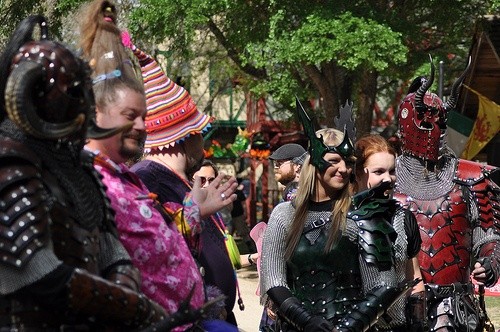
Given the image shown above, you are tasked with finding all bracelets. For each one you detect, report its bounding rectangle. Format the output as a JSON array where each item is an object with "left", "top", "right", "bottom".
[{"left": 248, "top": 254, "right": 257, "bottom": 266}]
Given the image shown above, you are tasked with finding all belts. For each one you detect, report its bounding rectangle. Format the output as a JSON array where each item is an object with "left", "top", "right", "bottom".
[{"left": 426, "top": 284, "right": 474, "bottom": 298}]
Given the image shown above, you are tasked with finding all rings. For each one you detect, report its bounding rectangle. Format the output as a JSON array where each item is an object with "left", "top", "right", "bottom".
[{"left": 221, "top": 193, "right": 228, "bottom": 200}]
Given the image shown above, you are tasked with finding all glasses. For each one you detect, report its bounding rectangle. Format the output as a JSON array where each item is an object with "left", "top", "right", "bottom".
[
  {"left": 192, "top": 177, "right": 215, "bottom": 185},
  {"left": 274, "top": 161, "right": 291, "bottom": 167}
]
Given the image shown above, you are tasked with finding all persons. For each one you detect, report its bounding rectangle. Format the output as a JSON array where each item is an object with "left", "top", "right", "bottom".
[
  {"left": 260, "top": 129, "right": 426, "bottom": 332},
  {"left": 82, "top": 72, "right": 260, "bottom": 332},
  {"left": 0, "top": 42, "right": 172, "bottom": 332},
  {"left": 356, "top": 89, "right": 500, "bottom": 332}
]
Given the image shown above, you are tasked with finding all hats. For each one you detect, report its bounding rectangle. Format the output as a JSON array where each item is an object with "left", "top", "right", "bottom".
[
  {"left": 122, "top": 29, "right": 216, "bottom": 153},
  {"left": 268, "top": 144, "right": 306, "bottom": 160}
]
